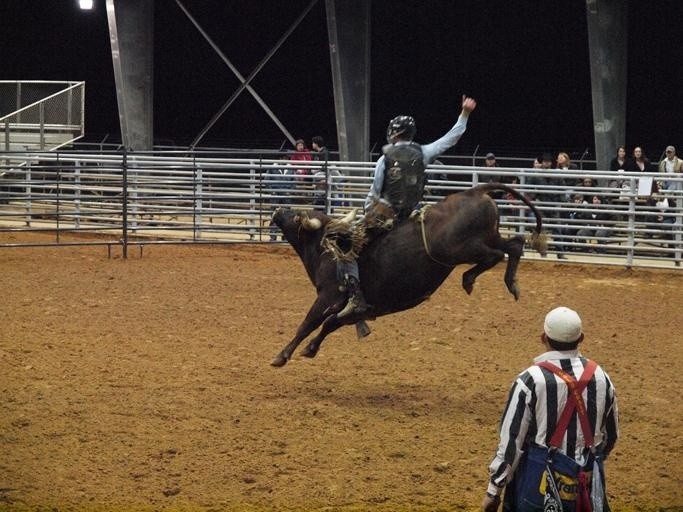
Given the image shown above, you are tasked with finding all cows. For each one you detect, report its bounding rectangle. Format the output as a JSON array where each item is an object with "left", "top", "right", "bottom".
[{"left": 268, "top": 181, "right": 549, "bottom": 369}]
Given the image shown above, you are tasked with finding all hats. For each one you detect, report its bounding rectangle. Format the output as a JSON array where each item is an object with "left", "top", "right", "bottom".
[
  {"left": 665, "top": 145, "right": 675, "bottom": 153},
  {"left": 486, "top": 152, "right": 495, "bottom": 160},
  {"left": 543, "top": 306, "right": 583, "bottom": 343}
]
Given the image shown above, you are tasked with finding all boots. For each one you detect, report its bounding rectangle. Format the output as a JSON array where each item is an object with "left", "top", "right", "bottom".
[{"left": 335, "top": 289, "right": 369, "bottom": 319}]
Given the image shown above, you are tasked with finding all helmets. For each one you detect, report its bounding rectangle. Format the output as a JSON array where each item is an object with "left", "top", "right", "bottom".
[{"left": 386, "top": 115, "right": 417, "bottom": 139}]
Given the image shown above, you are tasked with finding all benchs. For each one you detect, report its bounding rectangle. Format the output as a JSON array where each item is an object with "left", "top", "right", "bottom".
[{"left": 0, "top": 133, "right": 76, "bottom": 175}]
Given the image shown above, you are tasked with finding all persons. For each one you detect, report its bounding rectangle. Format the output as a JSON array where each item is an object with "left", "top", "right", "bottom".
[
  {"left": 426, "top": 159, "right": 448, "bottom": 201},
  {"left": 264, "top": 137, "right": 329, "bottom": 242},
  {"left": 479, "top": 305, "right": 624, "bottom": 511},
  {"left": 479, "top": 144, "right": 681, "bottom": 258},
  {"left": 317, "top": 93, "right": 481, "bottom": 322}
]
